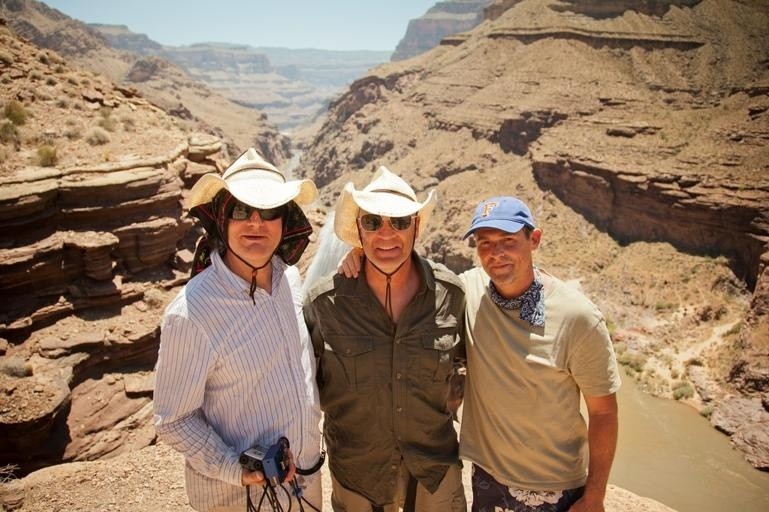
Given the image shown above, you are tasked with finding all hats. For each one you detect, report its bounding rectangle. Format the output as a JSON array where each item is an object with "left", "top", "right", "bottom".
[
  {"left": 462, "top": 197, "right": 535, "bottom": 241},
  {"left": 189, "top": 148, "right": 318, "bottom": 212},
  {"left": 334, "top": 166, "right": 437, "bottom": 249}
]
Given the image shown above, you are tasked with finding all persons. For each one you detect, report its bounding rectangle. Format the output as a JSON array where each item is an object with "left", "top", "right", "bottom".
[
  {"left": 336, "top": 196, "right": 621, "bottom": 512},
  {"left": 151, "top": 147, "right": 327, "bottom": 512},
  {"left": 302, "top": 166, "right": 467, "bottom": 512}
]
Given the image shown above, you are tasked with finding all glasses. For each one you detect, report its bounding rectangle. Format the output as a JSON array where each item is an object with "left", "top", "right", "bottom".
[
  {"left": 228, "top": 202, "right": 283, "bottom": 220},
  {"left": 357, "top": 214, "right": 418, "bottom": 232}
]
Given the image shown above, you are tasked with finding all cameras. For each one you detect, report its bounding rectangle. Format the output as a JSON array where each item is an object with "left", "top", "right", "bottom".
[
  {"left": 240, "top": 444, "right": 268, "bottom": 470},
  {"left": 263, "top": 436, "right": 290, "bottom": 485}
]
